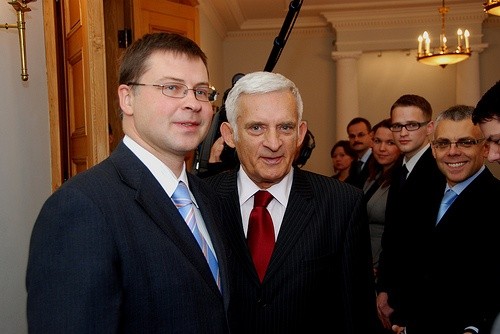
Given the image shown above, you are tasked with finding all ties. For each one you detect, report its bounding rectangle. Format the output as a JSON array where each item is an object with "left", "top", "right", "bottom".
[
  {"left": 172, "top": 182, "right": 223, "bottom": 292},
  {"left": 388, "top": 121, "right": 427, "bottom": 132},
  {"left": 356, "top": 161, "right": 363, "bottom": 172},
  {"left": 435, "top": 189, "right": 456, "bottom": 226},
  {"left": 401, "top": 164, "right": 409, "bottom": 182},
  {"left": 246, "top": 191, "right": 276, "bottom": 284}
]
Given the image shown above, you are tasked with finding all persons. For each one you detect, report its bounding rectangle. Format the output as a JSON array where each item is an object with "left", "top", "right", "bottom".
[
  {"left": 330, "top": 140, "right": 357, "bottom": 184},
  {"left": 377, "top": 105, "right": 500, "bottom": 334},
  {"left": 345, "top": 117, "right": 375, "bottom": 190},
  {"left": 363, "top": 119, "right": 402, "bottom": 284},
  {"left": 372, "top": 94, "right": 443, "bottom": 294},
  {"left": 474, "top": 82, "right": 500, "bottom": 334},
  {"left": 25, "top": 32, "right": 246, "bottom": 334},
  {"left": 199, "top": 71, "right": 379, "bottom": 334},
  {"left": 208, "top": 136, "right": 225, "bottom": 171}
]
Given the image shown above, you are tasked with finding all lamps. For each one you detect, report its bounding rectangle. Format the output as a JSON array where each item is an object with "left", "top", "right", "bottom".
[{"left": 416, "top": 0, "right": 471, "bottom": 70}]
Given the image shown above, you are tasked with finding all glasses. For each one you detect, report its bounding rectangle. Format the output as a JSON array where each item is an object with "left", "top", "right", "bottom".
[
  {"left": 434, "top": 139, "right": 479, "bottom": 151},
  {"left": 122, "top": 80, "right": 216, "bottom": 102}
]
